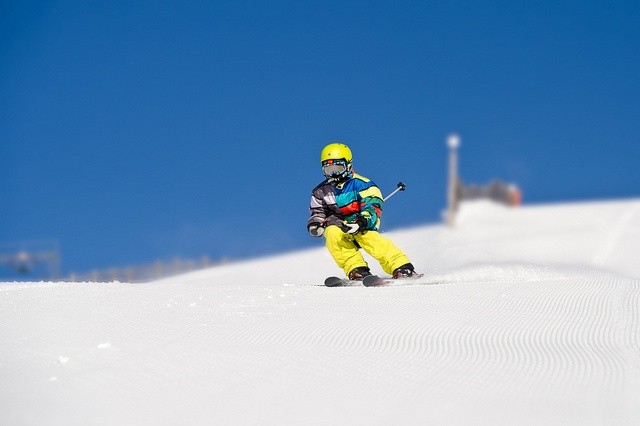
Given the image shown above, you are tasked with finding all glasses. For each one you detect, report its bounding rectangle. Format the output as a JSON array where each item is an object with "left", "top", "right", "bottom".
[{"left": 321, "top": 162, "right": 346, "bottom": 176}]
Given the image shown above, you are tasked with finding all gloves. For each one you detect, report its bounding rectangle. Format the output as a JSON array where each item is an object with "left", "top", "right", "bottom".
[
  {"left": 345, "top": 223, "right": 360, "bottom": 236},
  {"left": 306, "top": 222, "right": 325, "bottom": 238}
]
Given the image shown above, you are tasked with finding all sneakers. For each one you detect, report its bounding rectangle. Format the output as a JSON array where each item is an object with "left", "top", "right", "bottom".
[
  {"left": 349, "top": 271, "right": 372, "bottom": 281},
  {"left": 391, "top": 268, "right": 419, "bottom": 280}
]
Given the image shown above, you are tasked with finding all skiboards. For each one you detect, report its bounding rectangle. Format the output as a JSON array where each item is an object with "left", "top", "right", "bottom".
[{"left": 324, "top": 273, "right": 424, "bottom": 287}]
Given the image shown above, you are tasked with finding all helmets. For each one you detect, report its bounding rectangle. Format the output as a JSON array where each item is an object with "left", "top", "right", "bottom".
[{"left": 320, "top": 143, "right": 353, "bottom": 163}]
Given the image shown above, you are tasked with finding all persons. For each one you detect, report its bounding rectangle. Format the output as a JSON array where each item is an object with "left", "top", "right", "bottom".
[{"left": 306, "top": 143, "right": 418, "bottom": 277}]
